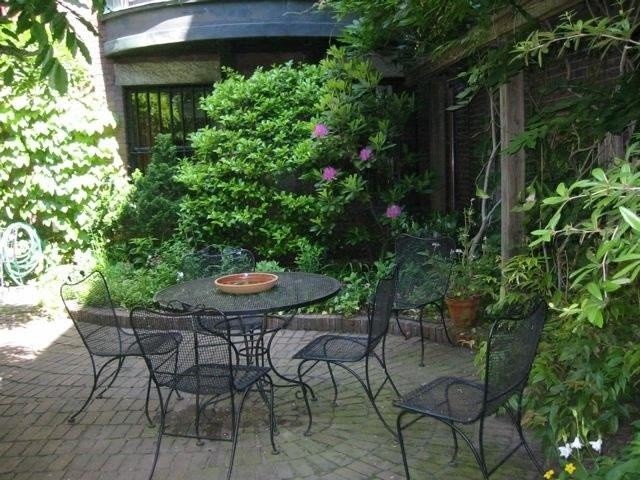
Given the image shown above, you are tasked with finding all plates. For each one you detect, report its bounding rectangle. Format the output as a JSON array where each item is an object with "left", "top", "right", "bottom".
[{"left": 214, "top": 273, "right": 278, "bottom": 294}]
